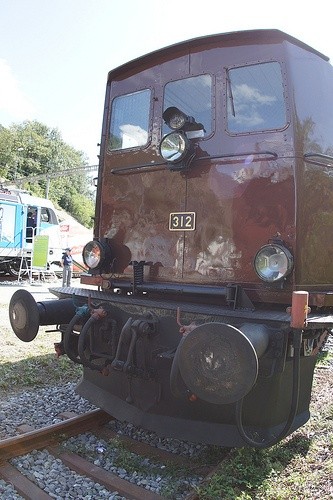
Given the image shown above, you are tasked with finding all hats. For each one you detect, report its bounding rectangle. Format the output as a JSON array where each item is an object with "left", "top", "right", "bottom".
[
  {"left": 65, "top": 248, "right": 72, "bottom": 251},
  {"left": 28, "top": 212, "right": 33, "bottom": 216}
]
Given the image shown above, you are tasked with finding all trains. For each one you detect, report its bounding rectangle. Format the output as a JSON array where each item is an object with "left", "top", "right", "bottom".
[
  {"left": 1, "top": 190, "right": 92, "bottom": 276},
  {"left": 10, "top": 29, "right": 333, "bottom": 446}
]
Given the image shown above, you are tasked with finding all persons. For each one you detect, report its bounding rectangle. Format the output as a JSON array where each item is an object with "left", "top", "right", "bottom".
[
  {"left": 26, "top": 211, "right": 35, "bottom": 243},
  {"left": 61, "top": 247, "right": 72, "bottom": 288}
]
